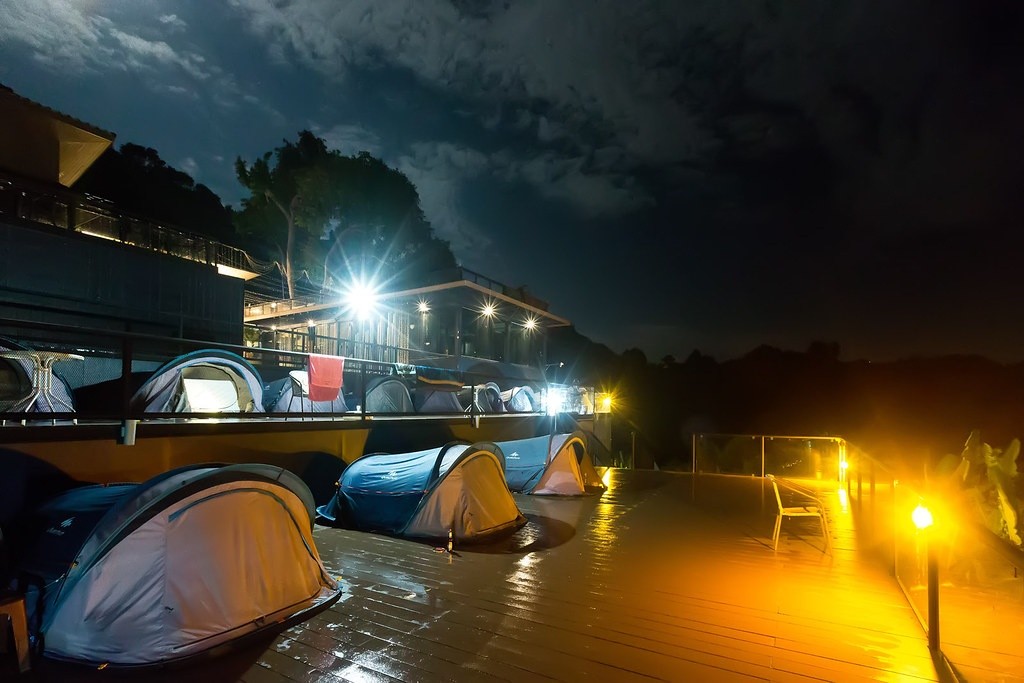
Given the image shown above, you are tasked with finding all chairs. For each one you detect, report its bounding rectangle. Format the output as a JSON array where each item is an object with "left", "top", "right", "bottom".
[
  {"left": 285, "top": 369, "right": 335, "bottom": 422},
  {"left": 765, "top": 472, "right": 834, "bottom": 555}
]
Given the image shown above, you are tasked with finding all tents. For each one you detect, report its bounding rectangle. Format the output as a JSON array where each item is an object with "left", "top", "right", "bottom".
[
  {"left": 361, "top": 367, "right": 590, "bottom": 416},
  {"left": 13, "top": 460, "right": 344, "bottom": 666},
  {"left": 1, "top": 333, "right": 76, "bottom": 423},
  {"left": 488, "top": 428, "right": 608, "bottom": 497},
  {"left": 266, "top": 374, "right": 352, "bottom": 412},
  {"left": 315, "top": 438, "right": 529, "bottom": 546},
  {"left": 117, "top": 347, "right": 267, "bottom": 419}
]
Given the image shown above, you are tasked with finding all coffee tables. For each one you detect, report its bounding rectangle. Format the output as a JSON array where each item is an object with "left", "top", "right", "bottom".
[{"left": 0, "top": 349, "right": 86, "bottom": 427}]
